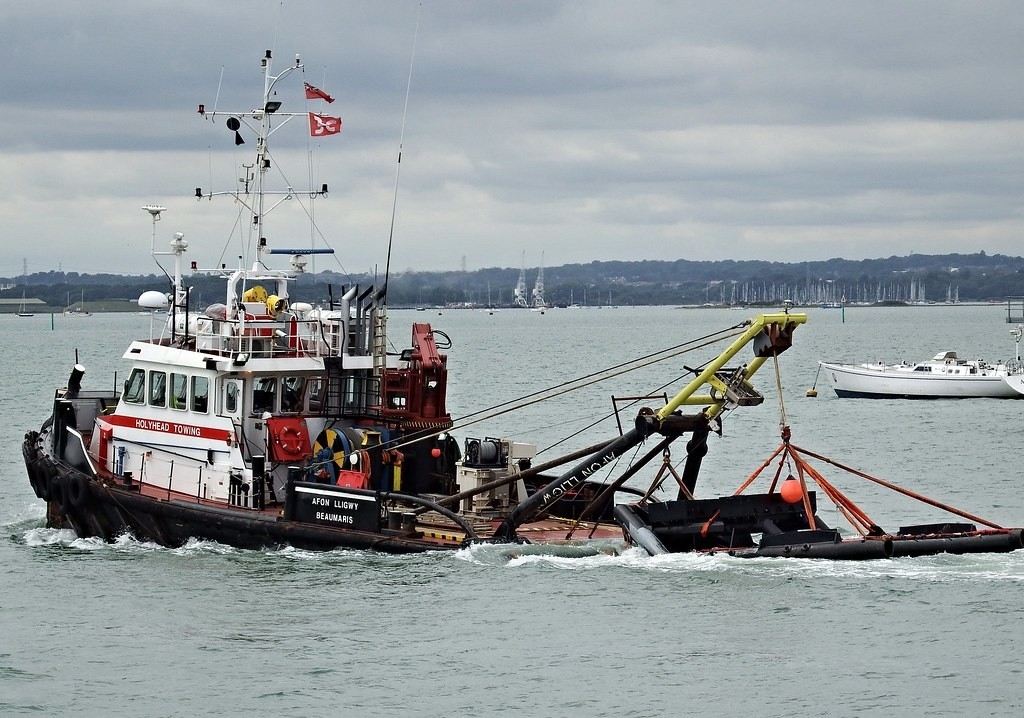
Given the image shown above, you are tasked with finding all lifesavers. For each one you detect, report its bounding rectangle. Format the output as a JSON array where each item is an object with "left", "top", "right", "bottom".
[
  {"left": 40, "top": 418, "right": 53, "bottom": 431},
  {"left": 279, "top": 425, "right": 304, "bottom": 454},
  {"left": 22, "top": 430, "right": 91, "bottom": 514}
]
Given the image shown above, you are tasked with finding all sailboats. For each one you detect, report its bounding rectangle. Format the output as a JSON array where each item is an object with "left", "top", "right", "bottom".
[{"left": 19, "top": 290, "right": 34, "bottom": 316}]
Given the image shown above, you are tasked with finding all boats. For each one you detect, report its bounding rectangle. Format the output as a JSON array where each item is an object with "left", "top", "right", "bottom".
[
  {"left": 138, "top": 307, "right": 166, "bottom": 316},
  {"left": 818, "top": 324, "right": 1024, "bottom": 398},
  {"left": 613, "top": 490, "right": 1024, "bottom": 562},
  {"left": 23, "top": 2, "right": 808, "bottom": 557},
  {"left": 63, "top": 289, "right": 93, "bottom": 317}
]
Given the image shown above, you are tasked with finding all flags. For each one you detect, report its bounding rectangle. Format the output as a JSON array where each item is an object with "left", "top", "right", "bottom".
[
  {"left": 309, "top": 112, "right": 342, "bottom": 136},
  {"left": 304, "top": 83, "right": 335, "bottom": 103}
]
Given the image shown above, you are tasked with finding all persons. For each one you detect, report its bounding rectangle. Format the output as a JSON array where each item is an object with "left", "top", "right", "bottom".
[
  {"left": 291, "top": 387, "right": 302, "bottom": 411},
  {"left": 161, "top": 386, "right": 179, "bottom": 408}
]
